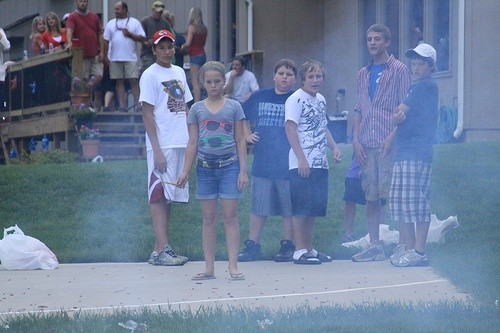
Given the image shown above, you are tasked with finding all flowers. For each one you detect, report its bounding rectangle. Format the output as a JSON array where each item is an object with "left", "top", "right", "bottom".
[
  {"left": 79, "top": 125, "right": 103, "bottom": 140},
  {"left": 70, "top": 104, "right": 95, "bottom": 119},
  {"left": 71, "top": 76, "right": 96, "bottom": 92}
]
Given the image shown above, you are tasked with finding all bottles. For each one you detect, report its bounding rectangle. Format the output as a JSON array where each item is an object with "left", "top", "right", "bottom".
[
  {"left": 39, "top": 42, "right": 45, "bottom": 55},
  {"left": 23, "top": 50, "right": 28, "bottom": 60},
  {"left": 48, "top": 42, "right": 53, "bottom": 54}
]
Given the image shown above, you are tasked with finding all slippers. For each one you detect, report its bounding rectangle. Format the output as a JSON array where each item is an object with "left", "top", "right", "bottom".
[
  {"left": 315, "top": 251, "right": 333, "bottom": 262},
  {"left": 294, "top": 252, "right": 322, "bottom": 265},
  {"left": 229, "top": 272, "right": 245, "bottom": 281},
  {"left": 191, "top": 272, "right": 216, "bottom": 280}
]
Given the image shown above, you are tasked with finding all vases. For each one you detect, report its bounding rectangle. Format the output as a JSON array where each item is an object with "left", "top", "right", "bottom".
[
  {"left": 80, "top": 140, "right": 101, "bottom": 157},
  {"left": 77, "top": 120, "right": 92, "bottom": 130},
  {"left": 70, "top": 92, "right": 90, "bottom": 107}
]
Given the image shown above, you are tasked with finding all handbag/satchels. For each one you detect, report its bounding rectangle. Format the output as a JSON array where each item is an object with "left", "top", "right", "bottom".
[{"left": 0, "top": 224, "right": 60, "bottom": 271}]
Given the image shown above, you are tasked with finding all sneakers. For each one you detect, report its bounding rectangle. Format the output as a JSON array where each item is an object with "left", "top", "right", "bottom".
[
  {"left": 390, "top": 244, "right": 410, "bottom": 261},
  {"left": 147, "top": 245, "right": 188, "bottom": 266},
  {"left": 391, "top": 249, "right": 428, "bottom": 267},
  {"left": 274, "top": 240, "right": 295, "bottom": 262},
  {"left": 351, "top": 244, "right": 386, "bottom": 262},
  {"left": 236, "top": 240, "right": 261, "bottom": 262}
]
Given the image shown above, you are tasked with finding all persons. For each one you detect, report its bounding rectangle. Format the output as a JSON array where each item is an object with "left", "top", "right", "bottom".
[
  {"left": 238, "top": 58, "right": 299, "bottom": 262},
  {"left": 29, "top": 13, "right": 70, "bottom": 57},
  {"left": 180, "top": 6, "right": 208, "bottom": 103},
  {"left": 65, "top": 0, "right": 116, "bottom": 114},
  {"left": 0, "top": 27, "right": 10, "bottom": 66},
  {"left": 388, "top": 43, "right": 439, "bottom": 268},
  {"left": 352, "top": 24, "right": 416, "bottom": 263},
  {"left": 103, "top": 1, "right": 149, "bottom": 112},
  {"left": 140, "top": 0, "right": 187, "bottom": 72},
  {"left": 343, "top": 151, "right": 388, "bottom": 239},
  {"left": 176, "top": 60, "right": 249, "bottom": 281},
  {"left": 139, "top": 29, "right": 194, "bottom": 266},
  {"left": 222, "top": 55, "right": 260, "bottom": 106},
  {"left": 282, "top": 59, "right": 342, "bottom": 265}
]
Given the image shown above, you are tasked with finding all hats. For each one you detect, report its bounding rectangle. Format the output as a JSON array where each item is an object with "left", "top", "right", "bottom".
[
  {"left": 151, "top": 1, "right": 164, "bottom": 13},
  {"left": 152, "top": 30, "right": 175, "bottom": 46},
  {"left": 405, "top": 42, "right": 437, "bottom": 63}
]
